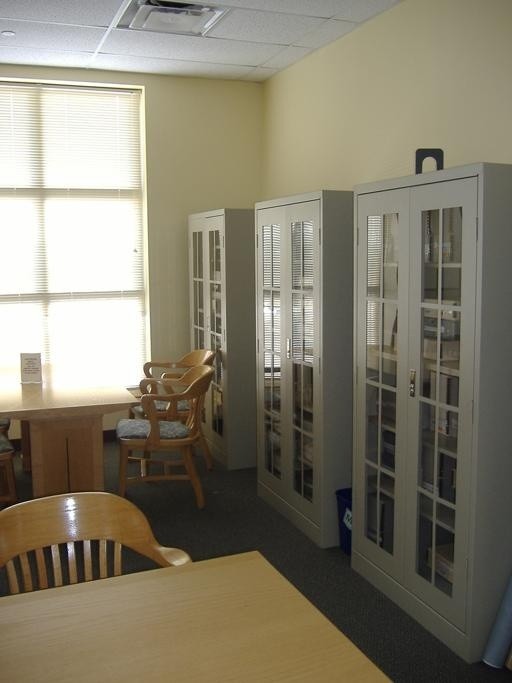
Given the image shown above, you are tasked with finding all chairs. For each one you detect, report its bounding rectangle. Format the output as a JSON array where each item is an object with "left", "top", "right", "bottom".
[
  {"left": 0, "top": 419, "right": 18, "bottom": 506},
  {"left": 116, "top": 349, "right": 217, "bottom": 509},
  {"left": 0, "top": 491, "right": 193, "bottom": 594}
]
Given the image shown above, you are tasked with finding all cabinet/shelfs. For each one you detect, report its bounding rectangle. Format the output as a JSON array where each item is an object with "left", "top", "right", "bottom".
[
  {"left": 254, "top": 191, "right": 354, "bottom": 549},
  {"left": 188, "top": 208, "right": 255, "bottom": 470},
  {"left": 351, "top": 162, "right": 512, "bottom": 666}
]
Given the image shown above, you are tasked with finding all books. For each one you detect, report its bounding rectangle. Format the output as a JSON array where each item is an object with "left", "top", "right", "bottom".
[
  {"left": 366, "top": 210, "right": 458, "bottom": 588},
  {"left": 265, "top": 349, "right": 316, "bottom": 499}
]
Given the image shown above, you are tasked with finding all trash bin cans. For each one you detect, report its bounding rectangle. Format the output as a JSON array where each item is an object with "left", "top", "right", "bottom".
[{"left": 335, "top": 487, "right": 352, "bottom": 557}]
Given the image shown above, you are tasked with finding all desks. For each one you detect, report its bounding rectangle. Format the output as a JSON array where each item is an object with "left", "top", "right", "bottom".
[
  {"left": 0, "top": 551, "right": 397, "bottom": 683},
  {"left": 0, "top": 363, "right": 141, "bottom": 499}
]
